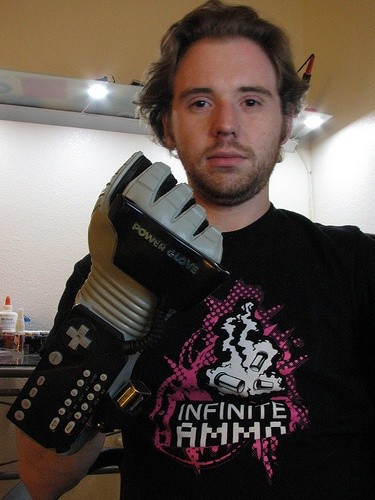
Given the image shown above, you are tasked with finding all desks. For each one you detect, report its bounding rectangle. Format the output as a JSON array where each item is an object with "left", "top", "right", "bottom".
[{"left": 0, "top": 331, "right": 53, "bottom": 406}]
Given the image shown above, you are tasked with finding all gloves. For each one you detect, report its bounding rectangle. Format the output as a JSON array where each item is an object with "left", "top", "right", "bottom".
[{"left": 73, "top": 151, "right": 230, "bottom": 341}]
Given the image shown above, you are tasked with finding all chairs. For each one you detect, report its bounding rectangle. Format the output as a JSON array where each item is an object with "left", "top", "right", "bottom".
[{"left": 0, "top": 428, "right": 126, "bottom": 500}]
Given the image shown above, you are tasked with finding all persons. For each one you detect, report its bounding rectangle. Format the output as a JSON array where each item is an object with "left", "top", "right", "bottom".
[{"left": 4, "top": 1, "right": 375, "bottom": 500}]
